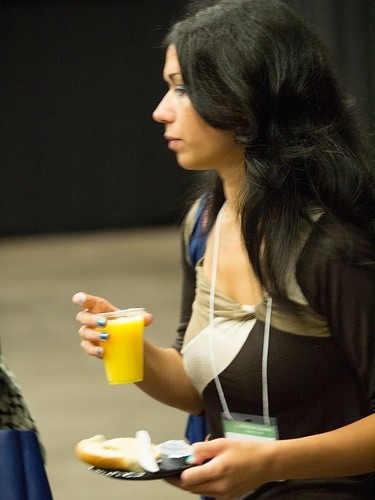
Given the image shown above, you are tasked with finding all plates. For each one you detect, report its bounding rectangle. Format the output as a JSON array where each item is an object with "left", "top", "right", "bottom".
[{"left": 90, "top": 454, "right": 210, "bottom": 479}]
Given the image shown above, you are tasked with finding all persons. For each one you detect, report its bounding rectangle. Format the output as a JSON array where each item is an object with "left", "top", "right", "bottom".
[{"left": 73, "top": 0, "right": 374, "bottom": 500}]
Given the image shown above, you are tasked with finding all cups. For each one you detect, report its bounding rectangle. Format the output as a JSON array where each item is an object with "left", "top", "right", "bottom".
[{"left": 96, "top": 306, "right": 144, "bottom": 385}]
